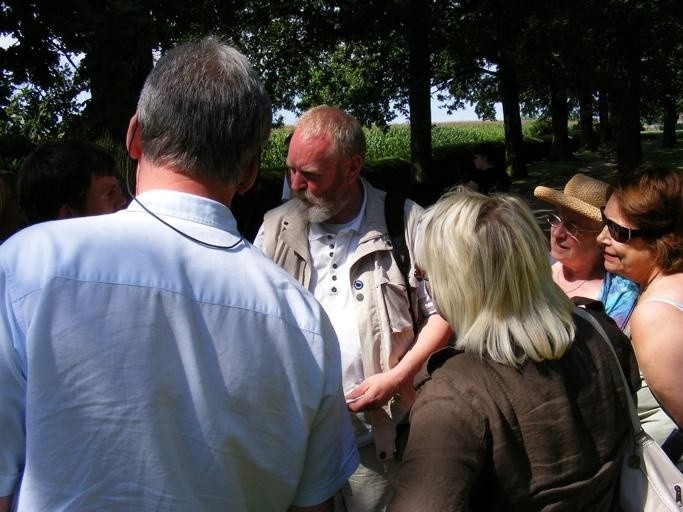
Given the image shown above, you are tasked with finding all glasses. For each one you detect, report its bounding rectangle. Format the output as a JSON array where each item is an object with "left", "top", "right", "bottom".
[
  {"left": 600, "top": 205, "right": 680, "bottom": 242},
  {"left": 545, "top": 212, "right": 600, "bottom": 237}
]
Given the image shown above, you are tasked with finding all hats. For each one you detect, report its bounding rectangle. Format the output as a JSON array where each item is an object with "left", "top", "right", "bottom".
[{"left": 533, "top": 173, "right": 617, "bottom": 225}]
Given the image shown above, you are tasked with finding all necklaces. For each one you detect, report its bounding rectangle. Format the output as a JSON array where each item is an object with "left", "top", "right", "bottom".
[{"left": 555, "top": 263, "right": 595, "bottom": 294}]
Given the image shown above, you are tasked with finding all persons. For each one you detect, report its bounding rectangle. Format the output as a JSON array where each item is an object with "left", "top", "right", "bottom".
[
  {"left": 278, "top": 130, "right": 294, "bottom": 203},
  {"left": 592, "top": 162, "right": 681, "bottom": 474},
  {"left": 532, "top": 173, "right": 640, "bottom": 337},
  {"left": 0, "top": 40, "right": 363, "bottom": 510},
  {"left": 15, "top": 137, "right": 127, "bottom": 226},
  {"left": 464, "top": 141, "right": 511, "bottom": 196},
  {"left": 384, "top": 184, "right": 644, "bottom": 512},
  {"left": 243, "top": 104, "right": 454, "bottom": 511},
  {"left": 0, "top": 170, "right": 28, "bottom": 244}
]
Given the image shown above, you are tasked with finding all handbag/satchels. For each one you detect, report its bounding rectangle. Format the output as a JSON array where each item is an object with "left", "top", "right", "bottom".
[{"left": 570, "top": 304, "right": 682, "bottom": 511}]
